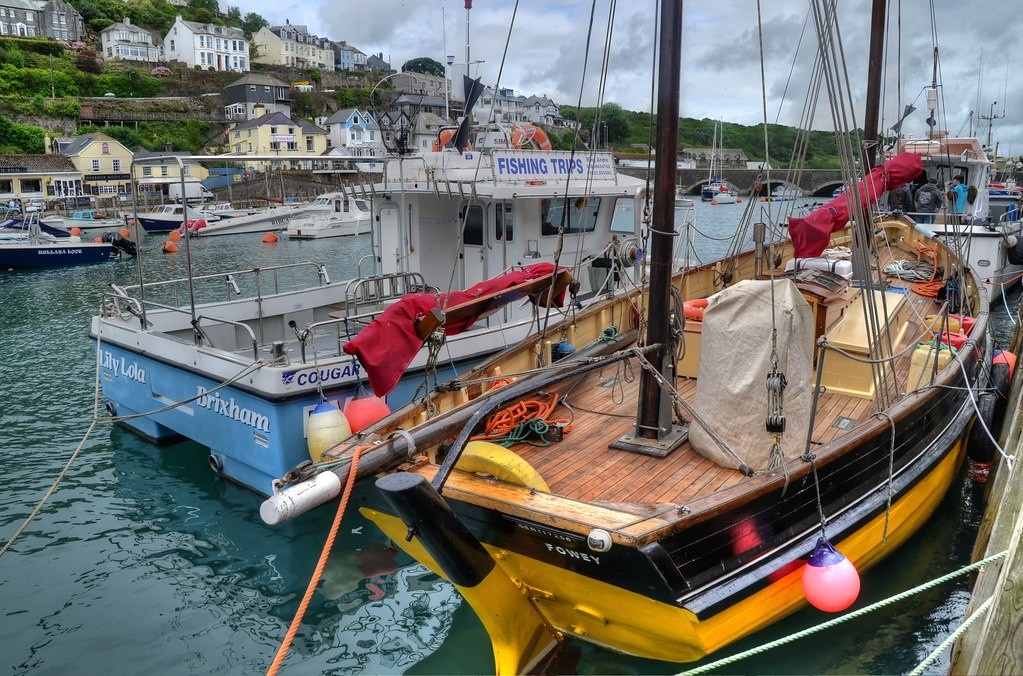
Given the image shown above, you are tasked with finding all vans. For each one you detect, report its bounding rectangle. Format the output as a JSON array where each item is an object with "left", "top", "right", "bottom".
[{"left": 169, "top": 182, "right": 214, "bottom": 203}]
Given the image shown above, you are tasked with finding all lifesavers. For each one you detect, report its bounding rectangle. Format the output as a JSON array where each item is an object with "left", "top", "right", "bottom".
[
  {"left": 969, "top": 394, "right": 998, "bottom": 460},
  {"left": 510, "top": 123, "right": 552, "bottom": 153},
  {"left": 432, "top": 129, "right": 471, "bottom": 151},
  {"left": 681, "top": 298, "right": 709, "bottom": 323},
  {"left": 990, "top": 362, "right": 1011, "bottom": 398}
]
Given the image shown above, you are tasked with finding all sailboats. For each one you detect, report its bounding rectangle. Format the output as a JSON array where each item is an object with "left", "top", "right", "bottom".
[{"left": 189, "top": 0, "right": 804, "bottom": 241}]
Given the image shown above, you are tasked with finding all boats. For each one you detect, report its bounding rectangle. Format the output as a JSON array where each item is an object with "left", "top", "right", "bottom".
[
  {"left": 88, "top": 5, "right": 698, "bottom": 504},
  {"left": 875, "top": 46, "right": 1023, "bottom": 302},
  {"left": 313, "top": 0, "right": 1016, "bottom": 676}
]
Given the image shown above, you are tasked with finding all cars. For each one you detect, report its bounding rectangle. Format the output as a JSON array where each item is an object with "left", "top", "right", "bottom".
[
  {"left": 0, "top": 202, "right": 21, "bottom": 216},
  {"left": 25, "top": 198, "right": 46, "bottom": 211},
  {"left": 0, "top": 196, "right": 256, "bottom": 267}
]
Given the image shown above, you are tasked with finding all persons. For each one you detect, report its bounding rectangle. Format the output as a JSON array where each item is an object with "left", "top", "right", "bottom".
[
  {"left": 915, "top": 179, "right": 942, "bottom": 224},
  {"left": 888, "top": 185, "right": 912, "bottom": 216},
  {"left": 947, "top": 175, "right": 967, "bottom": 225}
]
